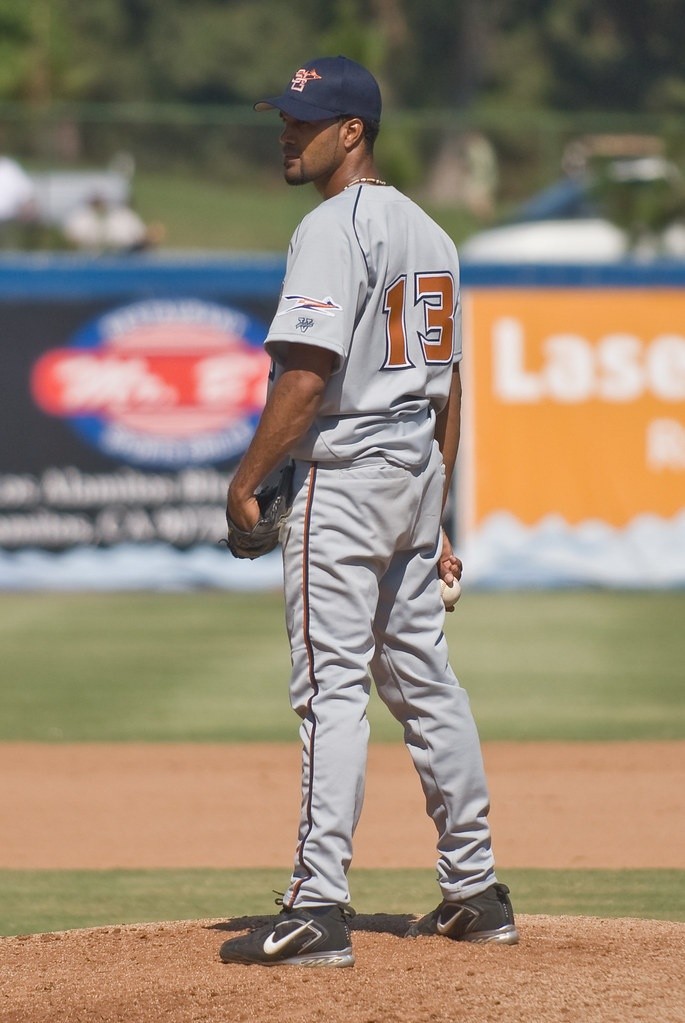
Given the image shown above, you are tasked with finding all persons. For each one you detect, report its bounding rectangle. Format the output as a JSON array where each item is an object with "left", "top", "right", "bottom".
[{"left": 214, "top": 56, "right": 521, "bottom": 966}]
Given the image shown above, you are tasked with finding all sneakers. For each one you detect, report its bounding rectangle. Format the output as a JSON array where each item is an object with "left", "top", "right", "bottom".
[
  {"left": 219, "top": 901, "right": 357, "bottom": 967},
  {"left": 405, "top": 882, "right": 516, "bottom": 944}
]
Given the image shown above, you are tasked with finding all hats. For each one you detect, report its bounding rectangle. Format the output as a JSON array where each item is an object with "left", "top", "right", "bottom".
[{"left": 253, "top": 55, "right": 382, "bottom": 122}]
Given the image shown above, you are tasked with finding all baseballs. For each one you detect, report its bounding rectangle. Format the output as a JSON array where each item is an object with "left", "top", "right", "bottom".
[{"left": 439, "top": 576, "right": 462, "bottom": 608}]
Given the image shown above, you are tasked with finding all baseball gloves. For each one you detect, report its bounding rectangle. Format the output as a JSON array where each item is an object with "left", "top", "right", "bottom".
[{"left": 217, "top": 463, "right": 296, "bottom": 562}]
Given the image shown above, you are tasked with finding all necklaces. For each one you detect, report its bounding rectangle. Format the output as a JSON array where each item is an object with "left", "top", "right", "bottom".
[{"left": 342, "top": 177, "right": 386, "bottom": 191}]
